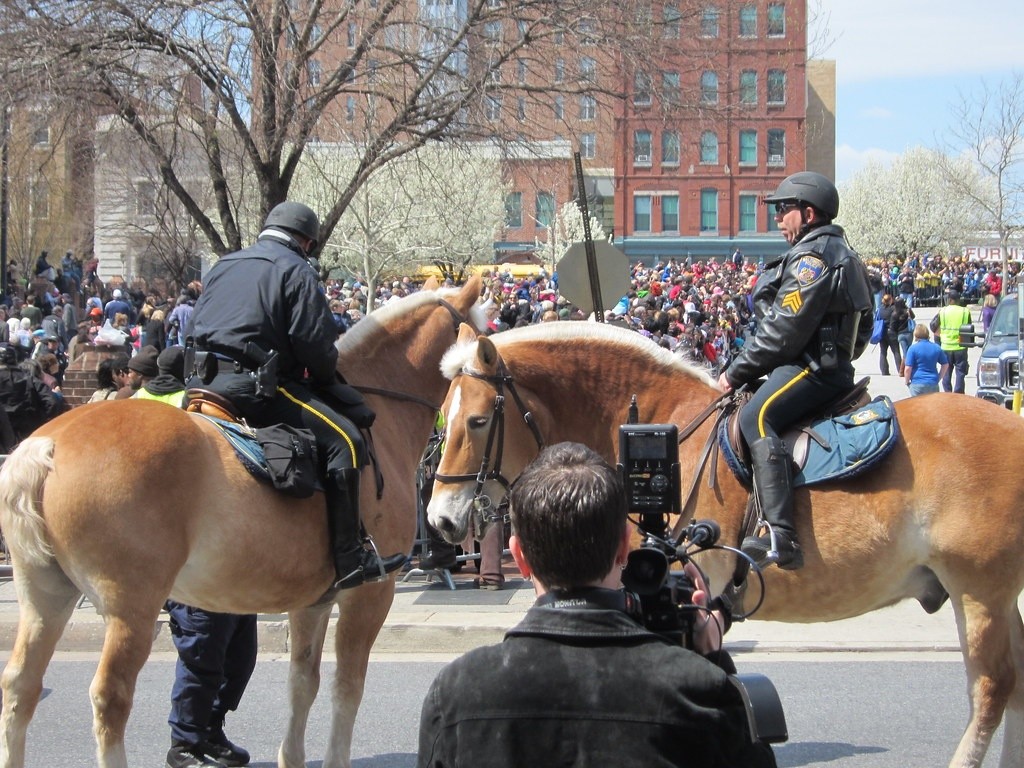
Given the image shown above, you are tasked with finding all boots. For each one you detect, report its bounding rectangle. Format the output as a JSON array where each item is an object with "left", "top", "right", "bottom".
[
  {"left": 899, "top": 359, "right": 905, "bottom": 377},
  {"left": 741, "top": 437, "right": 804, "bottom": 570},
  {"left": 324, "top": 466, "right": 408, "bottom": 590}
]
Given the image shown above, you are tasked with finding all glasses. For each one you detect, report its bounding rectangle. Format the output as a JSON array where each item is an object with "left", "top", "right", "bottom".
[{"left": 775, "top": 202, "right": 818, "bottom": 214}]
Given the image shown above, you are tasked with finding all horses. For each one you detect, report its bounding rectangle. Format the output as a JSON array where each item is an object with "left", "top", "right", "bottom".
[
  {"left": 426, "top": 321, "right": 1024, "bottom": 768},
  {"left": 0, "top": 270, "right": 484, "bottom": 768}
]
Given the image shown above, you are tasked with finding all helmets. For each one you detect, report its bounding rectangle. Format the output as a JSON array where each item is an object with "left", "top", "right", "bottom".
[
  {"left": 263, "top": 202, "right": 320, "bottom": 257},
  {"left": 763, "top": 171, "right": 839, "bottom": 219}
]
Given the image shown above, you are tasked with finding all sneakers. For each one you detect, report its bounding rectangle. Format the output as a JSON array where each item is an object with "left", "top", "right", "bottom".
[
  {"left": 418, "top": 553, "right": 457, "bottom": 570},
  {"left": 165, "top": 737, "right": 229, "bottom": 768},
  {"left": 203, "top": 730, "right": 250, "bottom": 767},
  {"left": 473, "top": 576, "right": 503, "bottom": 591}
]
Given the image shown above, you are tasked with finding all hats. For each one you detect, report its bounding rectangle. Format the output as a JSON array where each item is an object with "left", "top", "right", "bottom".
[
  {"left": 143, "top": 344, "right": 187, "bottom": 396},
  {"left": 127, "top": 344, "right": 159, "bottom": 376},
  {"left": 31, "top": 329, "right": 46, "bottom": 337},
  {"left": 48, "top": 334, "right": 60, "bottom": 344},
  {"left": 88, "top": 308, "right": 102, "bottom": 318},
  {"left": 882, "top": 295, "right": 893, "bottom": 306}
]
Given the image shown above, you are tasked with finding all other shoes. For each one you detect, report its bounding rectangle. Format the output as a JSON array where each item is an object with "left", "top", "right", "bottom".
[{"left": 882, "top": 371, "right": 890, "bottom": 376}]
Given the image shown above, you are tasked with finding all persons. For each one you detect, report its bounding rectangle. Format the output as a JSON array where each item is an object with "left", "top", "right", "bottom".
[
  {"left": 862, "top": 252, "right": 1024, "bottom": 395},
  {"left": 417, "top": 441, "right": 778, "bottom": 767},
  {"left": 0, "top": 249, "right": 201, "bottom": 456},
  {"left": 718, "top": 171, "right": 875, "bottom": 570},
  {"left": 326, "top": 245, "right": 766, "bottom": 591},
  {"left": 162, "top": 600, "right": 258, "bottom": 768},
  {"left": 182, "top": 202, "right": 408, "bottom": 590}
]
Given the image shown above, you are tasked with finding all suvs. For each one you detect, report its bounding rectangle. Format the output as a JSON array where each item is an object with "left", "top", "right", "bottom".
[{"left": 958, "top": 293, "right": 1024, "bottom": 410}]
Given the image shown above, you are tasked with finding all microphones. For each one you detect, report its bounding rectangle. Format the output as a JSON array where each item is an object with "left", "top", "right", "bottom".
[{"left": 691, "top": 519, "right": 720, "bottom": 549}]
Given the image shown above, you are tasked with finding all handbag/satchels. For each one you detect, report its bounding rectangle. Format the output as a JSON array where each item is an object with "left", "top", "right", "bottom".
[
  {"left": 907, "top": 308, "right": 916, "bottom": 333},
  {"left": 257, "top": 423, "right": 319, "bottom": 498},
  {"left": 870, "top": 308, "right": 885, "bottom": 344}
]
